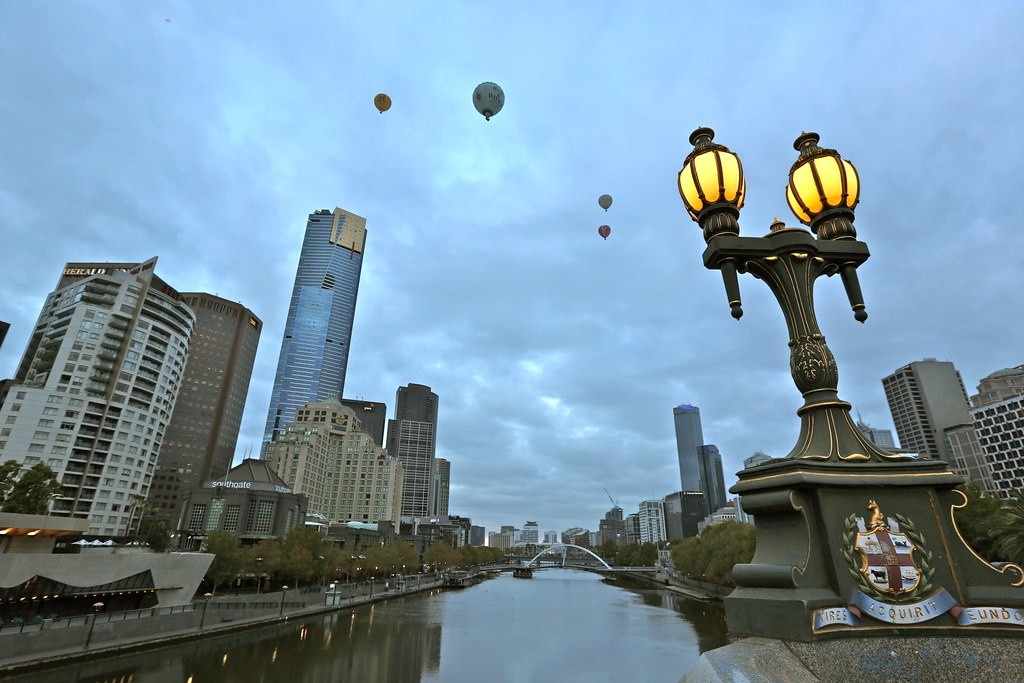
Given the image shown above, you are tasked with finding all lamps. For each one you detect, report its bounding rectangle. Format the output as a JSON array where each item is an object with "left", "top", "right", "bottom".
[
  {"left": 677, "top": 126, "right": 746, "bottom": 245},
  {"left": 785, "top": 131, "right": 861, "bottom": 241}
]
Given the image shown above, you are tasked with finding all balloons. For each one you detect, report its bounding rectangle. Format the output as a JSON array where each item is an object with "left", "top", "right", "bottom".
[
  {"left": 374, "top": 93, "right": 393, "bottom": 112},
  {"left": 598, "top": 225, "right": 612, "bottom": 238},
  {"left": 599, "top": 194, "right": 613, "bottom": 209},
  {"left": 473, "top": 81, "right": 506, "bottom": 117}
]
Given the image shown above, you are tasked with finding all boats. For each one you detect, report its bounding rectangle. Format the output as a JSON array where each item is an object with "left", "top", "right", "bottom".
[{"left": 512, "top": 567, "right": 534, "bottom": 578}]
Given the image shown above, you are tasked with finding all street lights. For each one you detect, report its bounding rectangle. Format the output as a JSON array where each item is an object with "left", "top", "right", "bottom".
[
  {"left": 678, "top": 125, "right": 1024, "bottom": 640},
  {"left": 369, "top": 577, "right": 375, "bottom": 598},
  {"left": 199, "top": 593, "right": 212, "bottom": 630},
  {"left": 83, "top": 602, "right": 105, "bottom": 649},
  {"left": 332, "top": 581, "right": 338, "bottom": 605},
  {"left": 279, "top": 586, "right": 288, "bottom": 615}
]
